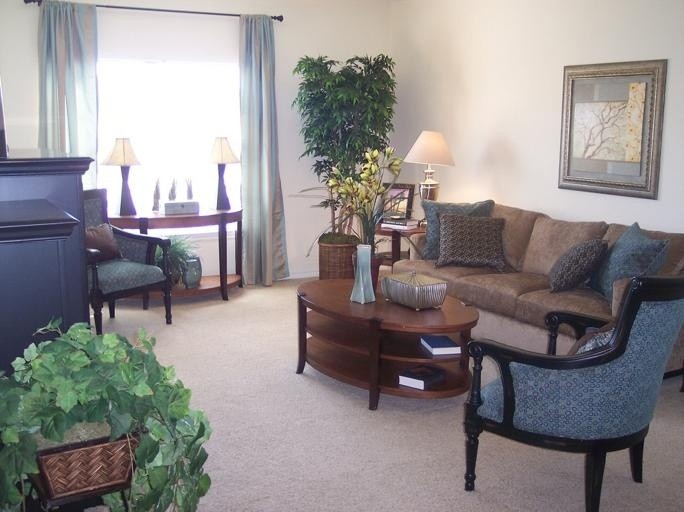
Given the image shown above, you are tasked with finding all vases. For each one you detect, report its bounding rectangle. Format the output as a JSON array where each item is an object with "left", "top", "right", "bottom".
[
  {"left": 182, "top": 256, "right": 202, "bottom": 289},
  {"left": 348, "top": 243, "right": 376, "bottom": 305},
  {"left": 350, "top": 252, "right": 384, "bottom": 293}
]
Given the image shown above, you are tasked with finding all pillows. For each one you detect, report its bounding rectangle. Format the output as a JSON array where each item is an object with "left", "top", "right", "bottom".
[
  {"left": 84, "top": 222, "right": 122, "bottom": 263},
  {"left": 586, "top": 220, "right": 671, "bottom": 308},
  {"left": 546, "top": 238, "right": 607, "bottom": 293},
  {"left": 434, "top": 210, "right": 508, "bottom": 274},
  {"left": 566, "top": 318, "right": 617, "bottom": 357},
  {"left": 419, "top": 199, "right": 494, "bottom": 260}
]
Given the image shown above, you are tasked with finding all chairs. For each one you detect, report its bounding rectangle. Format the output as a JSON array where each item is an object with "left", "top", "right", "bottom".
[
  {"left": 459, "top": 276, "right": 683, "bottom": 512},
  {"left": 82, "top": 187, "right": 171, "bottom": 336}
]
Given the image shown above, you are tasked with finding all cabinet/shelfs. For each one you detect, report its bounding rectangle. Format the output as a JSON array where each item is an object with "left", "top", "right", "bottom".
[
  {"left": 105, "top": 202, "right": 243, "bottom": 310},
  {"left": 0, "top": 147, "right": 96, "bottom": 379}
]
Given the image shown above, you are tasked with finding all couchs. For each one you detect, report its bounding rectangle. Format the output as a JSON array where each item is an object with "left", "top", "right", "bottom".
[{"left": 391, "top": 200, "right": 684, "bottom": 379}]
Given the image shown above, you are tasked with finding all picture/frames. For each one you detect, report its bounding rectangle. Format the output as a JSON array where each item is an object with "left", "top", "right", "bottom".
[
  {"left": 378, "top": 182, "right": 415, "bottom": 225},
  {"left": 557, "top": 58, "right": 668, "bottom": 200}
]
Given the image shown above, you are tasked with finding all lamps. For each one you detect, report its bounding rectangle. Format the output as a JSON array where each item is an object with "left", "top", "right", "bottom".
[
  {"left": 403, "top": 130, "right": 456, "bottom": 228},
  {"left": 101, "top": 137, "right": 141, "bottom": 216},
  {"left": 207, "top": 137, "right": 241, "bottom": 211}
]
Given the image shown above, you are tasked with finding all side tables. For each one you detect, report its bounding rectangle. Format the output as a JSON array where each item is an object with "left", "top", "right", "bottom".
[{"left": 367, "top": 217, "right": 426, "bottom": 279}]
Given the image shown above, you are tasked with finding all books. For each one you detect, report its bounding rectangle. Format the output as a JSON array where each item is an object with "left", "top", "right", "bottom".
[
  {"left": 382, "top": 215, "right": 418, "bottom": 226},
  {"left": 380, "top": 222, "right": 417, "bottom": 231},
  {"left": 420, "top": 335, "right": 461, "bottom": 356},
  {"left": 397, "top": 365, "right": 446, "bottom": 391}
]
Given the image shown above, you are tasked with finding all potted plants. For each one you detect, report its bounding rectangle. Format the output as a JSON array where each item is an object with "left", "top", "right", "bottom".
[
  {"left": 0, "top": 308, "right": 215, "bottom": 512},
  {"left": 150, "top": 234, "right": 200, "bottom": 286},
  {"left": 287, "top": 51, "right": 400, "bottom": 280}
]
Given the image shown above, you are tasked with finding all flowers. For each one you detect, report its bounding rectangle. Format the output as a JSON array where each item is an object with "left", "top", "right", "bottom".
[{"left": 298, "top": 147, "right": 426, "bottom": 257}]
